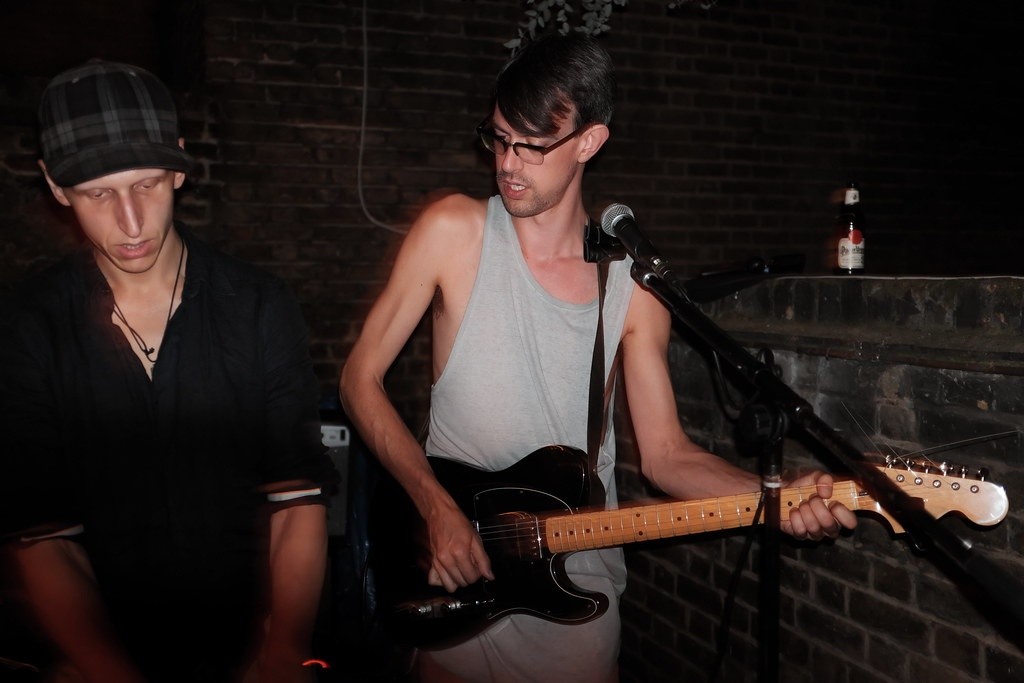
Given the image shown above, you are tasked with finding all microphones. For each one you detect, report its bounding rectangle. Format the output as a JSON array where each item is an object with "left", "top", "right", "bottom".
[{"left": 600, "top": 200, "right": 681, "bottom": 288}]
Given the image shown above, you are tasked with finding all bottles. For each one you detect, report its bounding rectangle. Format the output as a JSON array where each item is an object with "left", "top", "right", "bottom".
[
  {"left": 321, "top": 425, "right": 350, "bottom": 534},
  {"left": 835, "top": 182, "right": 865, "bottom": 275}
]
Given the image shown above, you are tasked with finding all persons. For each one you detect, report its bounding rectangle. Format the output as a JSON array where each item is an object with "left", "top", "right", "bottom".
[
  {"left": 339, "top": 33, "right": 859, "bottom": 683},
  {"left": 0, "top": 62, "right": 334, "bottom": 683}
]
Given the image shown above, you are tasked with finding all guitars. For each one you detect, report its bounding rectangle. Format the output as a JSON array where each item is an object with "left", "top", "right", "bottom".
[{"left": 357, "top": 442, "right": 1010, "bottom": 653}]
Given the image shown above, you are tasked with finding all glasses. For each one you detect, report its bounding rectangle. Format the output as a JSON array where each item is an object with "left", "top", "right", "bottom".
[{"left": 475, "top": 108, "right": 602, "bottom": 165}]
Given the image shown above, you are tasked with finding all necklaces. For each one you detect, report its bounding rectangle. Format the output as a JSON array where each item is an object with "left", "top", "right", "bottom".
[{"left": 113, "top": 238, "right": 184, "bottom": 363}]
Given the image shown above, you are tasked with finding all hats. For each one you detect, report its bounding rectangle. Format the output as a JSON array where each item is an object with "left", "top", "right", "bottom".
[{"left": 40, "top": 57, "right": 199, "bottom": 188}]
